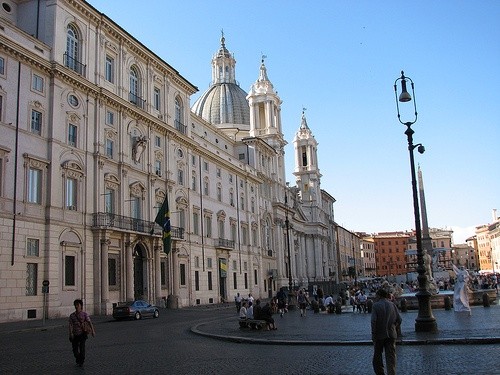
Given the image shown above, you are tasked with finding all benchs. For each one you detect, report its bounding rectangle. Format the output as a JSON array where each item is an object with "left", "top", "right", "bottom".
[{"left": 237, "top": 318, "right": 267, "bottom": 331}]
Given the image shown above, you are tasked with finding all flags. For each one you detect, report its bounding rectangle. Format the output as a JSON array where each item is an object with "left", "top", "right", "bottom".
[{"left": 155, "top": 194, "right": 172, "bottom": 256}]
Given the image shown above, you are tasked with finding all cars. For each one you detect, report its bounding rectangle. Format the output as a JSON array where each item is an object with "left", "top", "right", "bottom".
[{"left": 113, "top": 300, "right": 160, "bottom": 321}]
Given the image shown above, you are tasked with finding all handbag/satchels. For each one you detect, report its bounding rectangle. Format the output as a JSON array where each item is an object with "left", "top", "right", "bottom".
[
  {"left": 82, "top": 321, "right": 96, "bottom": 336},
  {"left": 396, "top": 321, "right": 400, "bottom": 337},
  {"left": 282, "top": 298, "right": 288, "bottom": 302}
]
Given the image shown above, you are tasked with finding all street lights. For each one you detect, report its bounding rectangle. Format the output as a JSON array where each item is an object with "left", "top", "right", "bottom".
[{"left": 393, "top": 70, "right": 440, "bottom": 331}]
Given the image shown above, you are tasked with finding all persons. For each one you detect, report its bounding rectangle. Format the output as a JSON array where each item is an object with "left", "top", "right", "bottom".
[
  {"left": 422, "top": 250, "right": 434, "bottom": 281},
  {"left": 371, "top": 290, "right": 402, "bottom": 375},
  {"left": 449, "top": 260, "right": 472, "bottom": 316},
  {"left": 69, "top": 299, "right": 95, "bottom": 367},
  {"left": 235, "top": 271, "right": 500, "bottom": 330},
  {"left": 220, "top": 296, "right": 225, "bottom": 306}
]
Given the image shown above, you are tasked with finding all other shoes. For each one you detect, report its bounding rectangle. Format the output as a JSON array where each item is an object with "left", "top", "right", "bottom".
[
  {"left": 280, "top": 314, "right": 283, "bottom": 318},
  {"left": 301, "top": 314, "right": 302, "bottom": 317},
  {"left": 80, "top": 361, "right": 84, "bottom": 367},
  {"left": 76, "top": 358, "right": 80, "bottom": 364}
]
